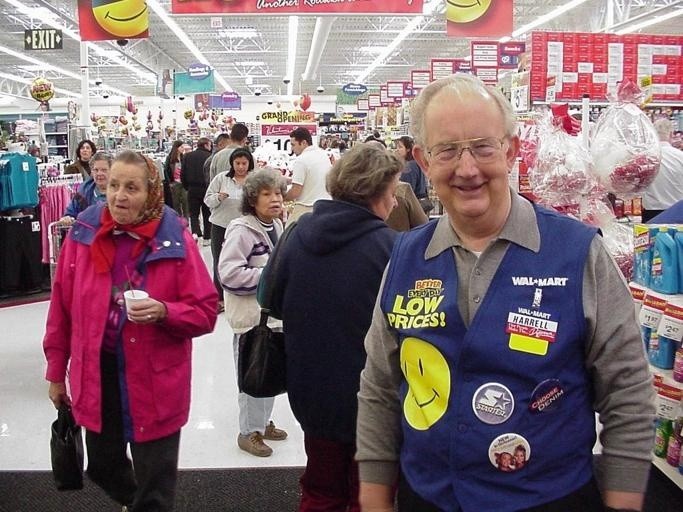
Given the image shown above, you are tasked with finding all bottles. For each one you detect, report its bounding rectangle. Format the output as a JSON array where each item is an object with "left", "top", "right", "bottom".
[
  {"left": 673, "top": 341, "right": 683, "bottom": 382},
  {"left": 652, "top": 417, "right": 672, "bottom": 457},
  {"left": 679, "top": 444, "right": 683, "bottom": 475},
  {"left": 666, "top": 416, "right": 683, "bottom": 467}
]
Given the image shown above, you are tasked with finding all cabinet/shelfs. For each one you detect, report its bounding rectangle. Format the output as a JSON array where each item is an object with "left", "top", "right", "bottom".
[{"left": 0, "top": 111, "right": 70, "bottom": 159}]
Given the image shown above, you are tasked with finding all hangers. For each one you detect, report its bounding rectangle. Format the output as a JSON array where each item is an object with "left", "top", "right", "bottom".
[
  {"left": 1, "top": 142, "right": 42, "bottom": 165},
  {"left": 42, "top": 172, "right": 84, "bottom": 187},
  {"left": 2, "top": 209, "right": 34, "bottom": 221}
]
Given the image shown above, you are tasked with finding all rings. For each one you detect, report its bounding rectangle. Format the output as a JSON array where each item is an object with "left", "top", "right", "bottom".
[{"left": 147, "top": 314, "right": 152, "bottom": 320}]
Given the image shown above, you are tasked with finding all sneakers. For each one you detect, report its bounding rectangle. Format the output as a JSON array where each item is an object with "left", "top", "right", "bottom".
[
  {"left": 191, "top": 232, "right": 198, "bottom": 244},
  {"left": 237, "top": 431, "right": 272, "bottom": 457},
  {"left": 202, "top": 238, "right": 210, "bottom": 246},
  {"left": 216, "top": 299, "right": 224, "bottom": 313},
  {"left": 258, "top": 421, "right": 287, "bottom": 441}
]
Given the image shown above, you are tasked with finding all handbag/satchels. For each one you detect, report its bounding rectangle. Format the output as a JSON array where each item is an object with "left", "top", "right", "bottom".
[
  {"left": 50, "top": 401, "right": 84, "bottom": 491},
  {"left": 237, "top": 325, "right": 287, "bottom": 397}
]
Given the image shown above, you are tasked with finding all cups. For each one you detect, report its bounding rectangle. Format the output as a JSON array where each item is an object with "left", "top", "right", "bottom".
[{"left": 124, "top": 290, "right": 149, "bottom": 322}]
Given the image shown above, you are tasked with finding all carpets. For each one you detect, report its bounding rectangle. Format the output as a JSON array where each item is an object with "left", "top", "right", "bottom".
[{"left": 0, "top": 453, "right": 683, "bottom": 512}]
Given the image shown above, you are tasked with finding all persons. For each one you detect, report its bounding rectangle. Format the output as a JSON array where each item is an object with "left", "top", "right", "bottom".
[
  {"left": 320, "top": 135, "right": 351, "bottom": 156},
  {"left": 216, "top": 167, "right": 287, "bottom": 456},
  {"left": 283, "top": 127, "right": 332, "bottom": 229},
  {"left": 164, "top": 135, "right": 231, "bottom": 247},
  {"left": 365, "top": 133, "right": 429, "bottom": 232},
  {"left": 58, "top": 153, "right": 114, "bottom": 228},
  {"left": 640, "top": 119, "right": 683, "bottom": 223},
  {"left": 354, "top": 72, "right": 657, "bottom": 511},
  {"left": 43, "top": 150, "right": 220, "bottom": 512},
  {"left": 204, "top": 124, "right": 257, "bottom": 315},
  {"left": 63, "top": 139, "right": 97, "bottom": 182},
  {"left": 258, "top": 144, "right": 403, "bottom": 509}
]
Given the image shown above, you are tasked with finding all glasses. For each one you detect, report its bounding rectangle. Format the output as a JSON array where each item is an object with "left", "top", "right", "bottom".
[{"left": 423, "top": 136, "right": 508, "bottom": 164}]
